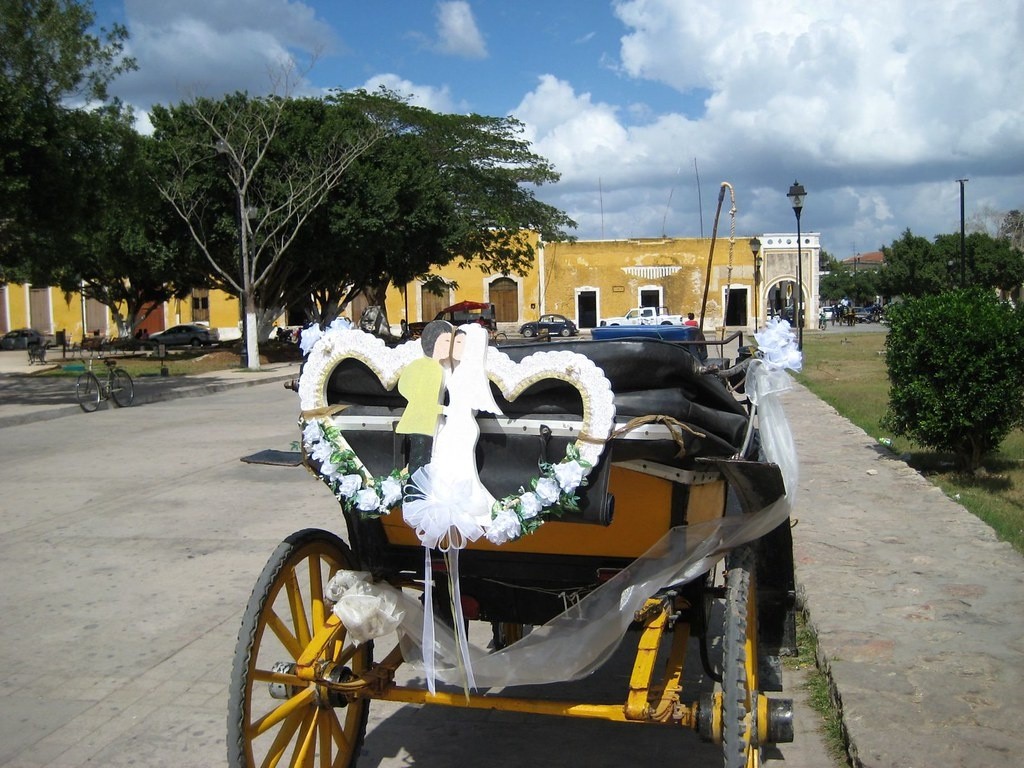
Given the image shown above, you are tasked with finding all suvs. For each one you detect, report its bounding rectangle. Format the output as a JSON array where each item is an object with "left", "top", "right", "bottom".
[{"left": 408, "top": 310, "right": 496, "bottom": 335}]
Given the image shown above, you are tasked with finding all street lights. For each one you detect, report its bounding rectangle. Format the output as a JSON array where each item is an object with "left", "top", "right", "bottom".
[
  {"left": 956, "top": 179, "right": 971, "bottom": 285},
  {"left": 785, "top": 178, "right": 808, "bottom": 353},
  {"left": 853, "top": 255, "right": 861, "bottom": 302},
  {"left": 749, "top": 238, "right": 762, "bottom": 332}
]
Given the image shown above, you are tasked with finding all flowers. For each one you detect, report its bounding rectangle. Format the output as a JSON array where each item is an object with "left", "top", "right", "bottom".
[
  {"left": 290, "top": 418, "right": 413, "bottom": 518},
  {"left": 484, "top": 441, "right": 593, "bottom": 546}
]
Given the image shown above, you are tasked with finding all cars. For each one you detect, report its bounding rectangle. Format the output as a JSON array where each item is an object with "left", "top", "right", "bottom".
[
  {"left": 767, "top": 302, "right": 886, "bottom": 323},
  {"left": 520, "top": 314, "right": 579, "bottom": 336},
  {"left": 149, "top": 322, "right": 219, "bottom": 349},
  {"left": 0, "top": 327, "right": 53, "bottom": 352}
]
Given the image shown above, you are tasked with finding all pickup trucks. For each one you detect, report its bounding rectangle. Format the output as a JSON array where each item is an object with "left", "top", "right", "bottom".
[{"left": 599, "top": 306, "right": 683, "bottom": 326}]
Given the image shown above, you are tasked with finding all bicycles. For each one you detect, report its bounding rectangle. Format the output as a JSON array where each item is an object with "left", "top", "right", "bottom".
[
  {"left": 487, "top": 327, "right": 508, "bottom": 347},
  {"left": 76, "top": 357, "right": 134, "bottom": 412}
]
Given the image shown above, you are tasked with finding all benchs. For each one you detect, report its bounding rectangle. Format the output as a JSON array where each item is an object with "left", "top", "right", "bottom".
[{"left": 27, "top": 335, "right": 130, "bottom": 366}]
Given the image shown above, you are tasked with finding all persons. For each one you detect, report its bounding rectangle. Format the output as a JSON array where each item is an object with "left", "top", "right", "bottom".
[
  {"left": 400, "top": 319, "right": 407, "bottom": 337},
  {"left": 874, "top": 303, "right": 880, "bottom": 307},
  {"left": 294, "top": 320, "right": 310, "bottom": 341},
  {"left": 685, "top": 313, "right": 698, "bottom": 328},
  {"left": 134, "top": 328, "right": 149, "bottom": 341},
  {"left": 841, "top": 296, "right": 849, "bottom": 310}
]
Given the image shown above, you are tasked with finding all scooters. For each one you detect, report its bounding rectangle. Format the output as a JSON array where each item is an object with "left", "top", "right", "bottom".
[
  {"left": 291, "top": 328, "right": 303, "bottom": 343},
  {"left": 819, "top": 313, "right": 826, "bottom": 330}
]
